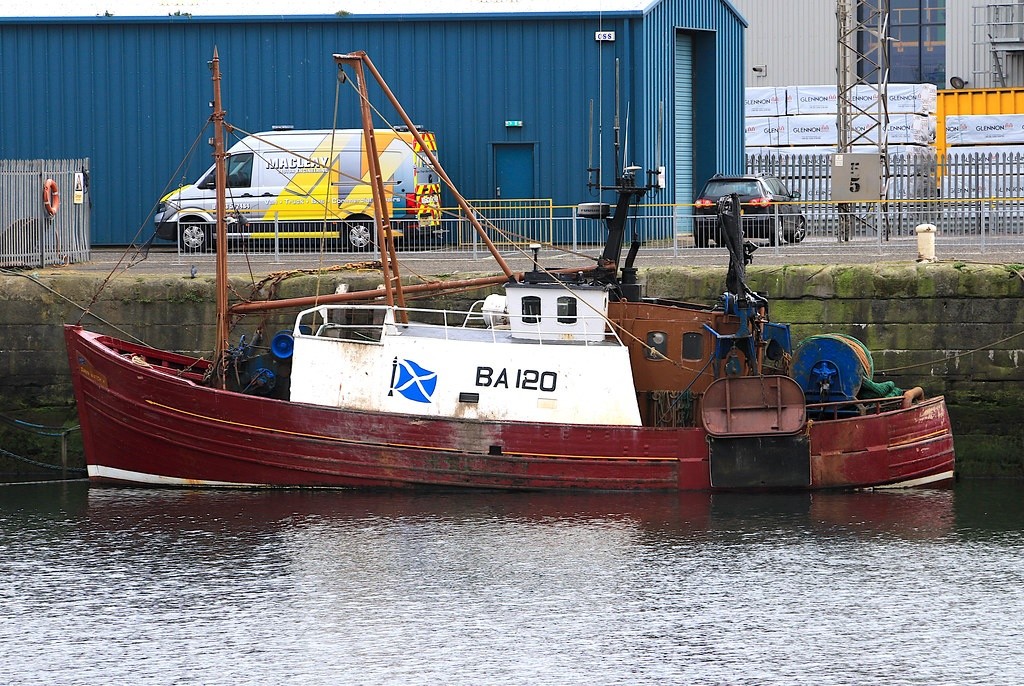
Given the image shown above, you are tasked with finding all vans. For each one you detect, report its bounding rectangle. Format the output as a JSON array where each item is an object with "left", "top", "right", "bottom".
[{"left": 152, "top": 122, "right": 443, "bottom": 251}]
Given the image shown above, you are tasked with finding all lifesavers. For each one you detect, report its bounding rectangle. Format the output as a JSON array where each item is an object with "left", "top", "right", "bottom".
[{"left": 44, "top": 179, "right": 61, "bottom": 215}]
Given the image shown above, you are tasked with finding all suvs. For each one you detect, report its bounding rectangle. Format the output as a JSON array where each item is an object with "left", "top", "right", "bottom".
[{"left": 691, "top": 171, "right": 808, "bottom": 245}]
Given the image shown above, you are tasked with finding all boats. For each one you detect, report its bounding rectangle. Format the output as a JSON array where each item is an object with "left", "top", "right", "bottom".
[{"left": 59, "top": 47, "right": 958, "bottom": 494}]
